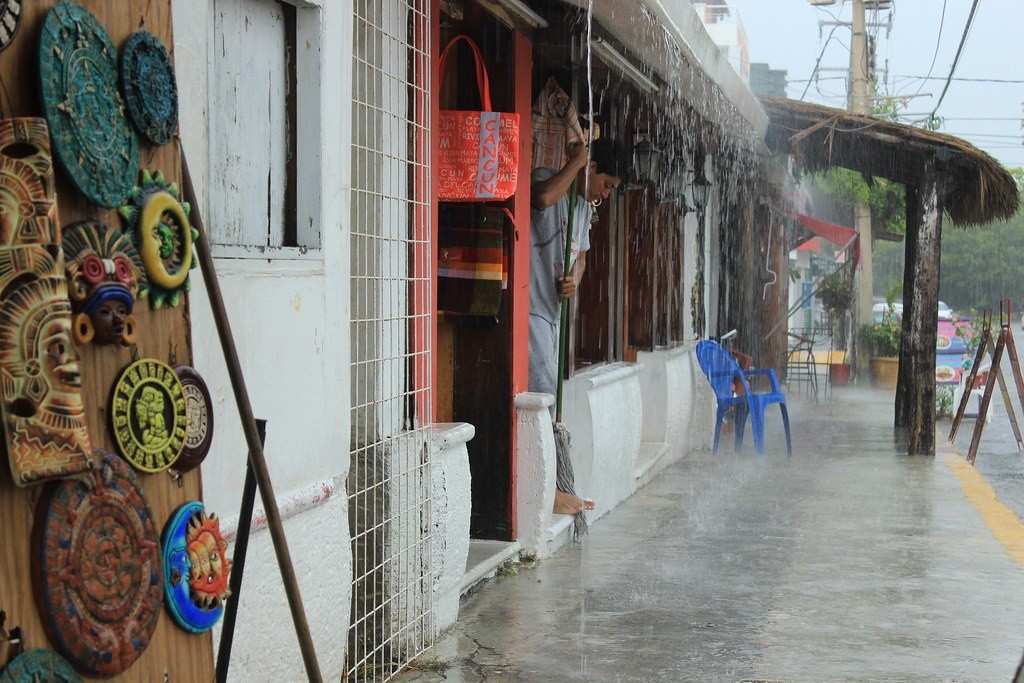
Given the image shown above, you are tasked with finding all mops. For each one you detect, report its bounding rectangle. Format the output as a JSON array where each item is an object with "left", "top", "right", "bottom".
[{"left": 549, "top": 174, "right": 589, "bottom": 545}]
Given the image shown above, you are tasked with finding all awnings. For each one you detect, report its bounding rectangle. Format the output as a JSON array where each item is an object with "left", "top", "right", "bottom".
[
  {"left": 759, "top": 93, "right": 1020, "bottom": 230},
  {"left": 795, "top": 213, "right": 861, "bottom": 266}
]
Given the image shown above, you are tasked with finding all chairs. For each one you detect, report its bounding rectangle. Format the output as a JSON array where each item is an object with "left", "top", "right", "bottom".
[{"left": 696, "top": 340, "right": 794, "bottom": 460}]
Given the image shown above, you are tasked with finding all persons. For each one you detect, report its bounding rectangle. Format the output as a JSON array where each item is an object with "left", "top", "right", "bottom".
[
  {"left": 78, "top": 277, "right": 135, "bottom": 345},
  {"left": 527, "top": 137, "right": 629, "bottom": 515}
]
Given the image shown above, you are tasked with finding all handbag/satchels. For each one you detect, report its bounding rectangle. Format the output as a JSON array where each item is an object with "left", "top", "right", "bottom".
[
  {"left": 432, "top": 206, "right": 509, "bottom": 323},
  {"left": 435, "top": 34, "right": 520, "bottom": 203},
  {"left": 527, "top": 70, "right": 585, "bottom": 173}
]
